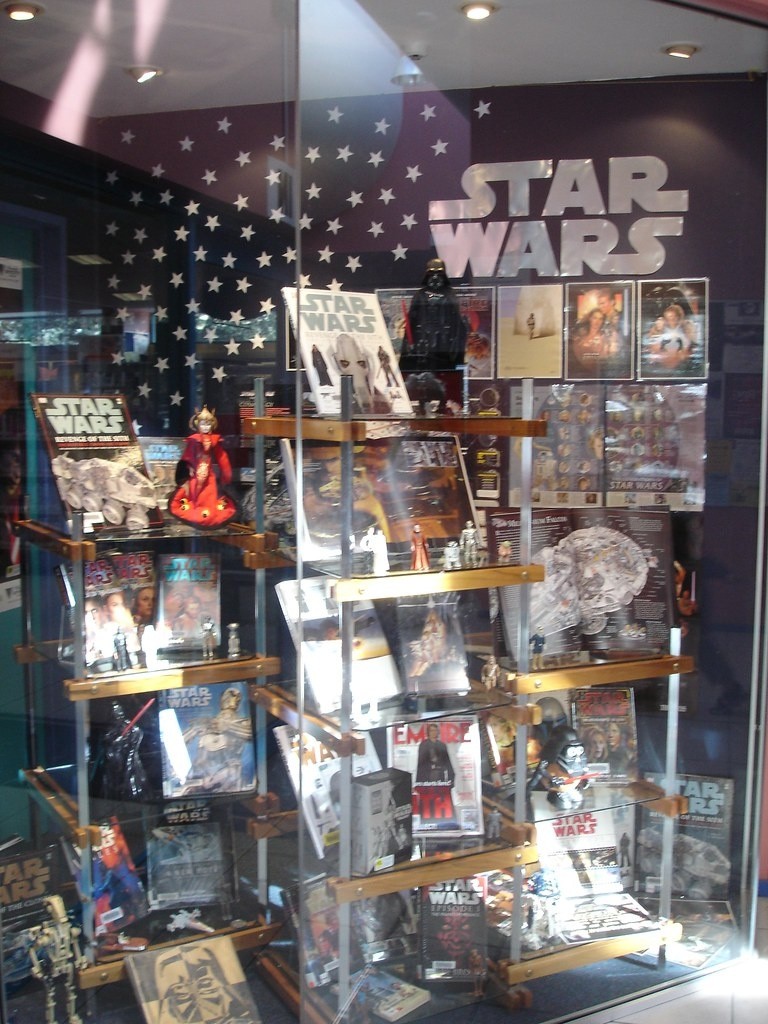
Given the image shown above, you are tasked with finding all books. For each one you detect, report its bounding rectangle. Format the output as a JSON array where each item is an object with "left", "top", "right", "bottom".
[
  {"left": 566, "top": 281, "right": 634, "bottom": 381},
  {"left": 278, "top": 427, "right": 479, "bottom": 561},
  {"left": 637, "top": 278, "right": 710, "bottom": 381},
  {"left": 82, "top": 502, "right": 732, "bottom": 1024},
  {"left": 496, "top": 285, "right": 562, "bottom": 379},
  {"left": 376, "top": 287, "right": 495, "bottom": 385},
  {"left": 279, "top": 284, "right": 414, "bottom": 418}
]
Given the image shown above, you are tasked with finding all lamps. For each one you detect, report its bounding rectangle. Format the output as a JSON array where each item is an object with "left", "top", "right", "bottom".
[
  {"left": 130, "top": 68, "right": 157, "bottom": 83},
  {"left": 461, "top": 3, "right": 491, "bottom": 20},
  {"left": 7, "top": 4, "right": 36, "bottom": 21},
  {"left": 666, "top": 46, "right": 695, "bottom": 58}
]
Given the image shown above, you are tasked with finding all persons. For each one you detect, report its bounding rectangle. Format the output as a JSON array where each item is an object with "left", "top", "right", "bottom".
[
  {"left": 398, "top": 258, "right": 466, "bottom": 378},
  {"left": 27, "top": 896, "right": 88, "bottom": 1024},
  {"left": 90, "top": 816, "right": 141, "bottom": 945},
  {"left": 410, "top": 524, "right": 432, "bottom": 571},
  {"left": 112, "top": 627, "right": 130, "bottom": 672},
  {"left": 486, "top": 805, "right": 503, "bottom": 846},
  {"left": 88, "top": 277, "right": 704, "bottom": 971},
  {"left": 535, "top": 722, "right": 589, "bottom": 809},
  {"left": 201, "top": 616, "right": 215, "bottom": 662},
  {"left": 529, "top": 626, "right": 546, "bottom": 671},
  {"left": 86, "top": 703, "right": 145, "bottom": 799},
  {"left": 459, "top": 520, "right": 478, "bottom": 568},
  {"left": 168, "top": 401, "right": 238, "bottom": 532},
  {"left": 479, "top": 653, "right": 501, "bottom": 705},
  {"left": 360, "top": 527, "right": 375, "bottom": 574},
  {"left": 371, "top": 529, "right": 390, "bottom": 574},
  {"left": 443, "top": 540, "right": 463, "bottom": 570}
]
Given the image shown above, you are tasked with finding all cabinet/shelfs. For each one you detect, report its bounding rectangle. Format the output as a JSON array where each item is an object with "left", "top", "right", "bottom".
[{"left": 0, "top": 341, "right": 761, "bottom": 1024}]
